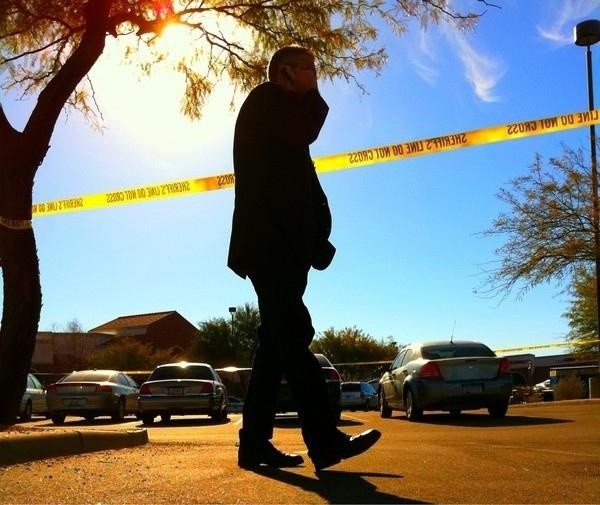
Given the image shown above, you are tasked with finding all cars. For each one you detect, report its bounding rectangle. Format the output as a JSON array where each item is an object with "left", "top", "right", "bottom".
[
  {"left": 274, "top": 353, "right": 342, "bottom": 421},
  {"left": 341, "top": 380, "right": 381, "bottom": 413},
  {"left": 136, "top": 362, "right": 229, "bottom": 426},
  {"left": 16, "top": 373, "right": 51, "bottom": 423},
  {"left": 372, "top": 334, "right": 540, "bottom": 424},
  {"left": 45, "top": 368, "right": 140, "bottom": 424}
]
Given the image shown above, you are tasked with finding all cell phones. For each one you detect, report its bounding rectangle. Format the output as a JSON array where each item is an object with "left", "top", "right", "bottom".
[{"left": 283, "top": 65, "right": 292, "bottom": 75}]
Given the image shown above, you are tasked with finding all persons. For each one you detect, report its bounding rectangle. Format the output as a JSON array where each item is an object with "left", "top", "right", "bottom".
[{"left": 227, "top": 45, "right": 381, "bottom": 471}]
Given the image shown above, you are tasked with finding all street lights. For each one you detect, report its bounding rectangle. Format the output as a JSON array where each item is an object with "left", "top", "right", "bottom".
[
  {"left": 227, "top": 306, "right": 237, "bottom": 354},
  {"left": 573, "top": 17, "right": 598, "bottom": 329}
]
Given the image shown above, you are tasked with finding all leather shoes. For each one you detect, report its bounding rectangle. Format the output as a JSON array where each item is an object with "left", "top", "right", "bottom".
[
  {"left": 313, "top": 429, "right": 382, "bottom": 470},
  {"left": 237, "top": 441, "right": 304, "bottom": 468}
]
